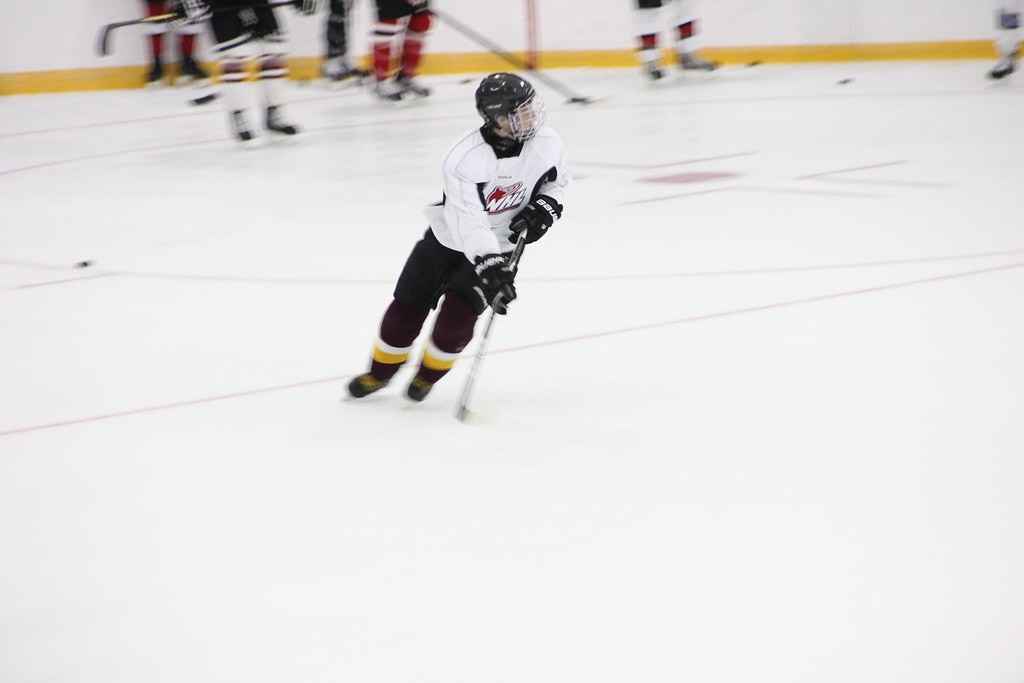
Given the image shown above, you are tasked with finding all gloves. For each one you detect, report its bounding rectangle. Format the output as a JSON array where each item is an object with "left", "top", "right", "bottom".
[
  {"left": 474, "top": 254, "right": 516, "bottom": 315},
  {"left": 170, "top": 2, "right": 186, "bottom": 19},
  {"left": 508, "top": 194, "right": 563, "bottom": 245}
]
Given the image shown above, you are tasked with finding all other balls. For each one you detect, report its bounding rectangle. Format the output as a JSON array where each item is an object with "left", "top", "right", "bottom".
[{"left": 77, "top": 261, "right": 92, "bottom": 268}]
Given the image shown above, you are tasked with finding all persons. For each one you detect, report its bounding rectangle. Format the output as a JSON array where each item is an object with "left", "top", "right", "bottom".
[
  {"left": 637, "top": 0, "right": 715, "bottom": 78},
  {"left": 347, "top": 73, "right": 563, "bottom": 404},
  {"left": 988, "top": 0, "right": 1024, "bottom": 78},
  {"left": 145, "top": 0, "right": 434, "bottom": 140}
]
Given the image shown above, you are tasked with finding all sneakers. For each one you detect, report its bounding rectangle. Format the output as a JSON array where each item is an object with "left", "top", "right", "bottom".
[
  {"left": 263, "top": 105, "right": 298, "bottom": 140},
  {"left": 228, "top": 110, "right": 254, "bottom": 141},
  {"left": 373, "top": 74, "right": 428, "bottom": 105}
]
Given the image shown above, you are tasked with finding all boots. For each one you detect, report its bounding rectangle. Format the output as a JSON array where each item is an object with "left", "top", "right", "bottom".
[
  {"left": 348, "top": 372, "right": 389, "bottom": 398},
  {"left": 408, "top": 373, "right": 433, "bottom": 401}
]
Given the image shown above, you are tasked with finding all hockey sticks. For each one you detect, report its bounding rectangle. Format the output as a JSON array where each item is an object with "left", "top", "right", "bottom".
[
  {"left": 430, "top": 9, "right": 612, "bottom": 106},
  {"left": 452, "top": 228, "right": 528, "bottom": 427},
  {"left": 98, "top": 0, "right": 294, "bottom": 56}
]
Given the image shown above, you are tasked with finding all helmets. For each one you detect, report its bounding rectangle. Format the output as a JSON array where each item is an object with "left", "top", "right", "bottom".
[{"left": 475, "top": 72, "right": 536, "bottom": 116}]
